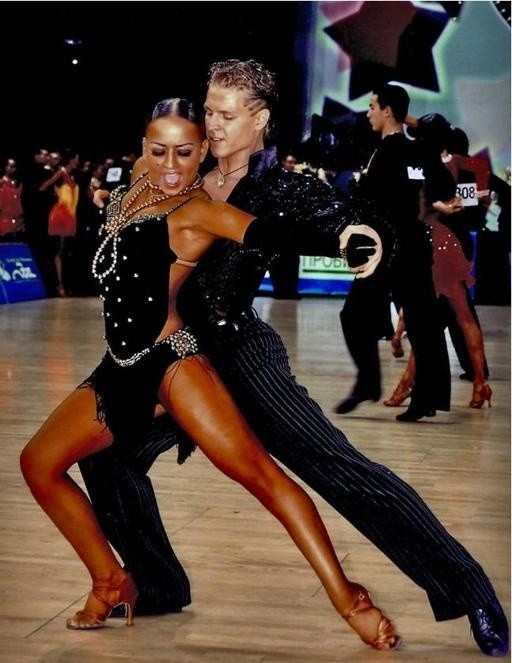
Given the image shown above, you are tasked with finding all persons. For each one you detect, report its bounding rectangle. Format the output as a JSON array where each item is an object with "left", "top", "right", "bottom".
[
  {"left": 275, "top": 84, "right": 493, "bottom": 422},
  {"left": 0, "top": 150, "right": 138, "bottom": 298},
  {"left": 77, "top": 59, "right": 509, "bottom": 656},
  {"left": 19, "top": 96, "right": 402, "bottom": 652}
]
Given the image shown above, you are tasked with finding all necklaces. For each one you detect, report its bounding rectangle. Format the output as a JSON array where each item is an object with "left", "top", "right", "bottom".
[
  {"left": 216, "top": 164, "right": 248, "bottom": 188},
  {"left": 90, "top": 173, "right": 204, "bottom": 280}
]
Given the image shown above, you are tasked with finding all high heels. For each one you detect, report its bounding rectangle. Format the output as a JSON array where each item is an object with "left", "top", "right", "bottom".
[
  {"left": 392, "top": 337, "right": 403, "bottom": 357},
  {"left": 334, "top": 581, "right": 401, "bottom": 652},
  {"left": 384, "top": 382, "right": 414, "bottom": 406},
  {"left": 67, "top": 574, "right": 138, "bottom": 630},
  {"left": 470, "top": 383, "right": 492, "bottom": 408}
]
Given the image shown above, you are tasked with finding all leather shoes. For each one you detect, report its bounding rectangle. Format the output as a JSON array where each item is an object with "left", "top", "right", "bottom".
[
  {"left": 336, "top": 385, "right": 381, "bottom": 414},
  {"left": 396, "top": 401, "right": 435, "bottom": 422},
  {"left": 466, "top": 598, "right": 509, "bottom": 656},
  {"left": 460, "top": 370, "right": 489, "bottom": 382},
  {"left": 110, "top": 591, "right": 182, "bottom": 617}
]
